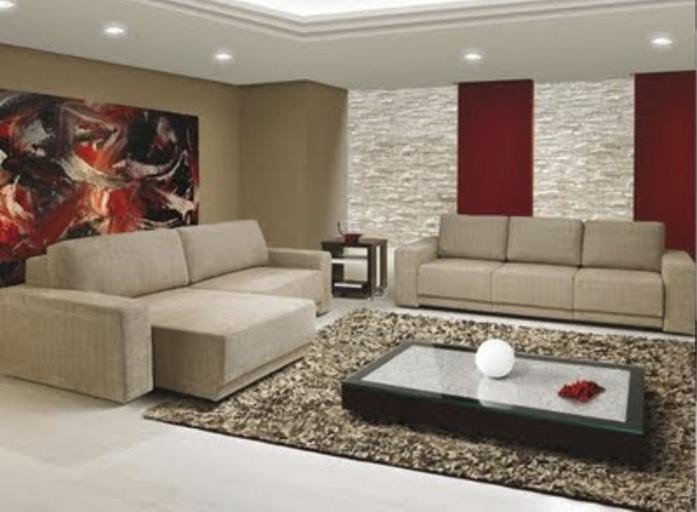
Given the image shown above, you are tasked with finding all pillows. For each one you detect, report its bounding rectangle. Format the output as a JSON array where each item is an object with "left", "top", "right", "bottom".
[
  {"left": 582, "top": 220, "right": 665, "bottom": 273},
  {"left": 505, "top": 215, "right": 585, "bottom": 267},
  {"left": 177, "top": 220, "right": 271, "bottom": 284},
  {"left": 436, "top": 213, "right": 508, "bottom": 265},
  {"left": 46, "top": 227, "right": 188, "bottom": 298}
]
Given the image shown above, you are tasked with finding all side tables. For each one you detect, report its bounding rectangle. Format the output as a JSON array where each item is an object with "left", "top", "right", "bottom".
[{"left": 323, "top": 238, "right": 387, "bottom": 301}]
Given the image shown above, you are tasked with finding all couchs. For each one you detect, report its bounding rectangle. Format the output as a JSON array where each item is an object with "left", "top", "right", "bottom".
[
  {"left": 0, "top": 219, "right": 332, "bottom": 404},
  {"left": 394, "top": 213, "right": 697, "bottom": 336}
]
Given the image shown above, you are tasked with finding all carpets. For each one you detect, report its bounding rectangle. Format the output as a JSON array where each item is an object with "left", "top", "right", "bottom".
[{"left": 142, "top": 307, "right": 697, "bottom": 512}]
